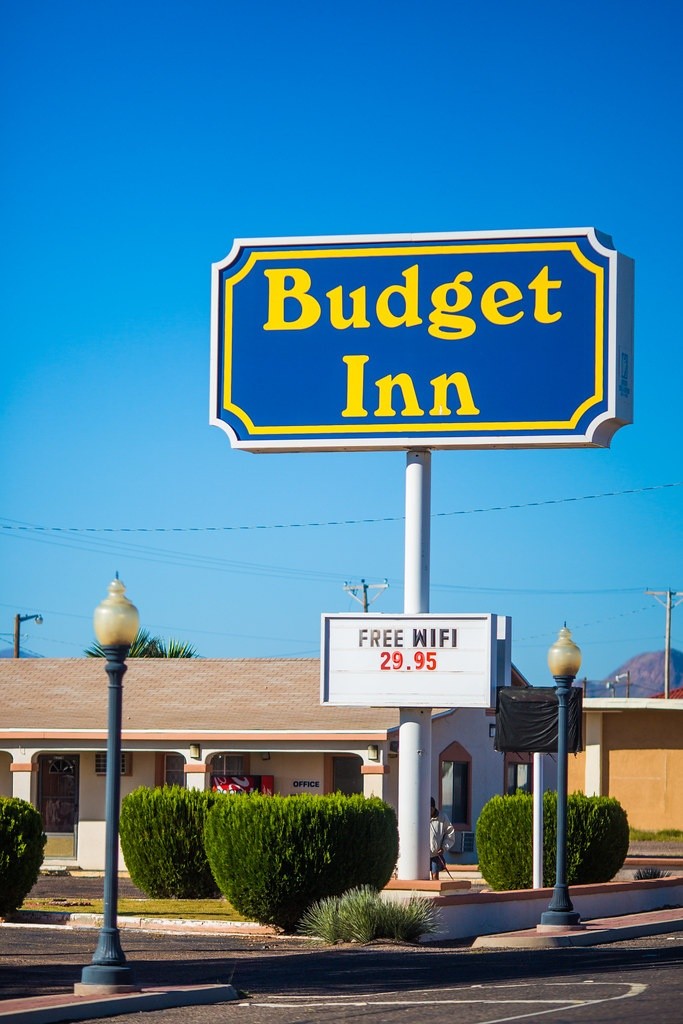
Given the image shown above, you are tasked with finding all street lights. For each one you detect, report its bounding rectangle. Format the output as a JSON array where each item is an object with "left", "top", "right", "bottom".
[
  {"left": 72, "top": 567, "right": 151, "bottom": 996},
  {"left": 13, "top": 612, "right": 45, "bottom": 658},
  {"left": 533, "top": 618, "right": 587, "bottom": 936}
]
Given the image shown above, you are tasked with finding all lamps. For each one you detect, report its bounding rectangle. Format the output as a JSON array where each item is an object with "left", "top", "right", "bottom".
[
  {"left": 189, "top": 744, "right": 201, "bottom": 758},
  {"left": 368, "top": 744, "right": 378, "bottom": 761}
]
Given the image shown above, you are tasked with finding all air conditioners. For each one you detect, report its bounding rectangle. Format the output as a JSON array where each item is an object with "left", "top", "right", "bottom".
[{"left": 450, "top": 830, "right": 475, "bottom": 853}]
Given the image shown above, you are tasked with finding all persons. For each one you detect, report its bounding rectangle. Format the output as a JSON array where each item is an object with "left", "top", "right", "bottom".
[{"left": 430, "top": 797, "right": 456, "bottom": 880}]
